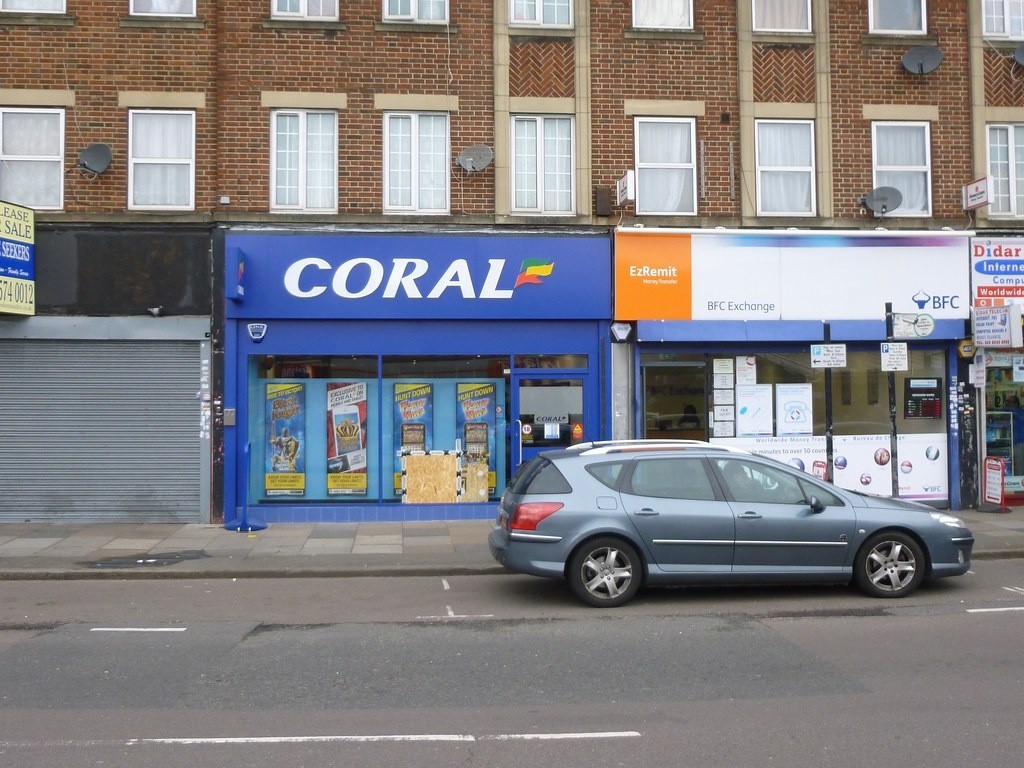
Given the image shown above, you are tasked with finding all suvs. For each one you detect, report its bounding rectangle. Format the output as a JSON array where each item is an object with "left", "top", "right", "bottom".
[{"left": 490, "top": 436, "right": 974, "bottom": 611}]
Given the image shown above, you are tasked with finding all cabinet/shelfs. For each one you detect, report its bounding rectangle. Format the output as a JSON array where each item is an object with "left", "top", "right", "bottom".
[{"left": 986, "top": 411, "right": 1014, "bottom": 476}]
